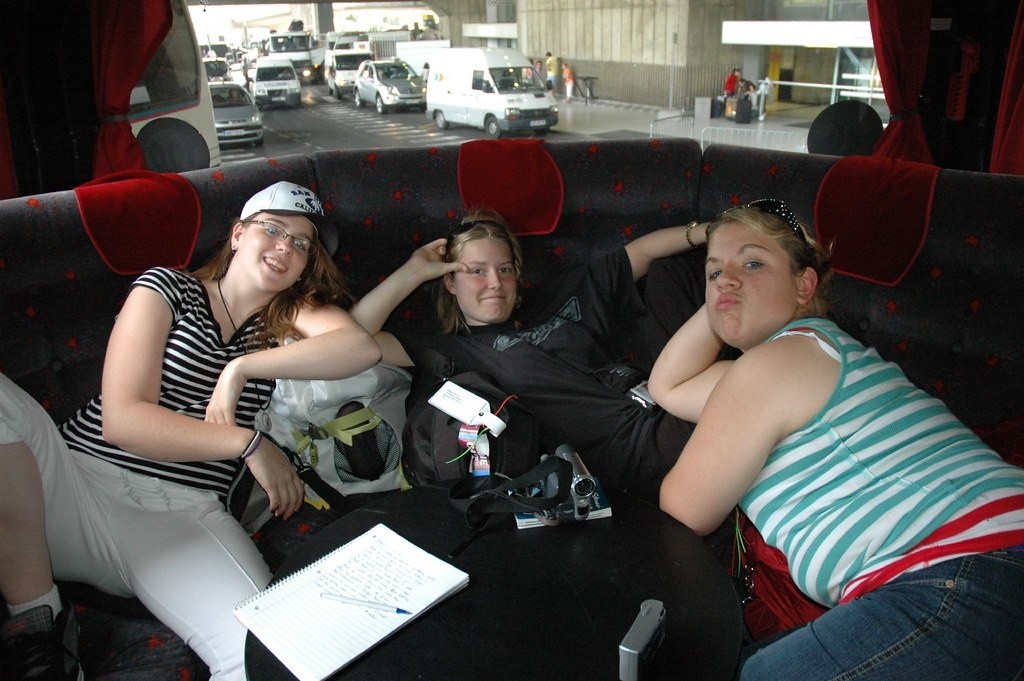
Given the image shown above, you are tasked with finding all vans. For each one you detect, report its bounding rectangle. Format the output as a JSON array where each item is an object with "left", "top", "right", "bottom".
[{"left": 252, "top": 55, "right": 302, "bottom": 105}]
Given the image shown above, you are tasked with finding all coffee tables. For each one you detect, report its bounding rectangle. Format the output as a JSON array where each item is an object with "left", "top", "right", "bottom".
[{"left": 245, "top": 479, "right": 743, "bottom": 681}]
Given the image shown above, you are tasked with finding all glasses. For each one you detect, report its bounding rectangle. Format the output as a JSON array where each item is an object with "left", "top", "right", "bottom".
[
  {"left": 242, "top": 221, "right": 317, "bottom": 256},
  {"left": 705, "top": 198, "right": 808, "bottom": 246},
  {"left": 446, "top": 220, "right": 509, "bottom": 243}
]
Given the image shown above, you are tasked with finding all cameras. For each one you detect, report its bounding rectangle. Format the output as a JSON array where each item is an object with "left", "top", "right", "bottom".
[
  {"left": 538, "top": 443, "right": 596, "bottom": 522},
  {"left": 618, "top": 597, "right": 667, "bottom": 681}
]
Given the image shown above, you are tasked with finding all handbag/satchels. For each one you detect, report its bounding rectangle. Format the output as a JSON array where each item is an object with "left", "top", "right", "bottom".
[
  {"left": 728, "top": 512, "right": 830, "bottom": 644},
  {"left": 405, "top": 370, "right": 575, "bottom": 533},
  {"left": 253, "top": 361, "right": 411, "bottom": 496}
]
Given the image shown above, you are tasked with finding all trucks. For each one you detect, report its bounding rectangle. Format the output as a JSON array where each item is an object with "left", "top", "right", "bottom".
[
  {"left": 268, "top": 31, "right": 326, "bottom": 87},
  {"left": 324, "top": 48, "right": 374, "bottom": 100},
  {"left": 425, "top": 47, "right": 559, "bottom": 139}
]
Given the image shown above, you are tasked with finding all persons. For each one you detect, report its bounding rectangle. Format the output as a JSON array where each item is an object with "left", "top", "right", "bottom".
[
  {"left": 390, "top": 68, "right": 405, "bottom": 78},
  {"left": 349, "top": 205, "right": 710, "bottom": 505},
  {"left": 647, "top": 197, "right": 1024, "bottom": 681},
  {"left": 0, "top": 178, "right": 383, "bottom": 681},
  {"left": 278, "top": 68, "right": 290, "bottom": 77},
  {"left": 562, "top": 63, "right": 574, "bottom": 102},
  {"left": 747, "top": 85, "right": 757, "bottom": 108},
  {"left": 230, "top": 90, "right": 243, "bottom": 101},
  {"left": 545, "top": 52, "right": 557, "bottom": 93},
  {"left": 240, "top": 57, "right": 250, "bottom": 91},
  {"left": 724, "top": 69, "right": 741, "bottom": 104},
  {"left": 536, "top": 61, "right": 543, "bottom": 75}
]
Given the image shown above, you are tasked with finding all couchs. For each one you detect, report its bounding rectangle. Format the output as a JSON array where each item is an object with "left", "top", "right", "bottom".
[{"left": 0, "top": 138, "right": 1024, "bottom": 680}]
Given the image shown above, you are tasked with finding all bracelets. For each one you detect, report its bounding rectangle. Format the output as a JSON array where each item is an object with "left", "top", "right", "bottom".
[{"left": 240, "top": 431, "right": 260, "bottom": 458}]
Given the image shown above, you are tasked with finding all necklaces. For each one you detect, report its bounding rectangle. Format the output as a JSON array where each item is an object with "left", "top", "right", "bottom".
[{"left": 218, "top": 277, "right": 273, "bottom": 432}]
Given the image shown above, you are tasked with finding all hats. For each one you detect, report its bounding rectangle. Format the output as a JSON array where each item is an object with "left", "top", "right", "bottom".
[{"left": 240, "top": 181, "right": 324, "bottom": 233}]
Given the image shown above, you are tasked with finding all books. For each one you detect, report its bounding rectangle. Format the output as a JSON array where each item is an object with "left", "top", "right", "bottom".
[
  {"left": 514, "top": 477, "right": 613, "bottom": 529},
  {"left": 233, "top": 522, "right": 469, "bottom": 681}
]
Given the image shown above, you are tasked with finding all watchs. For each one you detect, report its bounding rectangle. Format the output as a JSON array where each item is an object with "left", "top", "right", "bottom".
[{"left": 686, "top": 221, "right": 699, "bottom": 248}]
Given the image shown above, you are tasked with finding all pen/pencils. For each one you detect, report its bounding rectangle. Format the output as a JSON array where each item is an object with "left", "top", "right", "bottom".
[{"left": 321, "top": 592, "right": 412, "bottom": 615}]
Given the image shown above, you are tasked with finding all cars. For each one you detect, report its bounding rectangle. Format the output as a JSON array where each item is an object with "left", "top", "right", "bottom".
[
  {"left": 327, "top": 30, "right": 370, "bottom": 52},
  {"left": 352, "top": 56, "right": 429, "bottom": 115},
  {"left": 202, "top": 57, "right": 233, "bottom": 82},
  {"left": 207, "top": 81, "right": 264, "bottom": 151}
]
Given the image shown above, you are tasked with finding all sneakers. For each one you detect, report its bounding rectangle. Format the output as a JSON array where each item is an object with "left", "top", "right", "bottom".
[{"left": 0, "top": 598, "right": 84, "bottom": 681}]
